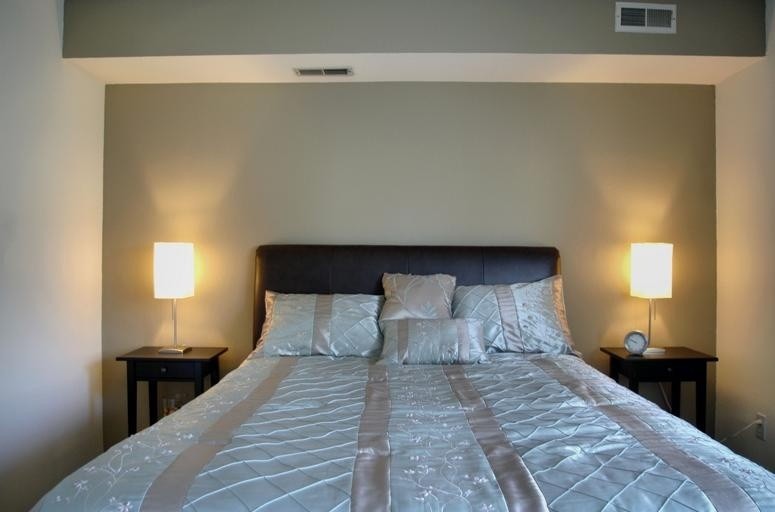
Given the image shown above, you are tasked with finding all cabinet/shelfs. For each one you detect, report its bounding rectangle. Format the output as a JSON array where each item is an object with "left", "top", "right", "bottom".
[{"left": 116, "top": 347, "right": 230, "bottom": 437}]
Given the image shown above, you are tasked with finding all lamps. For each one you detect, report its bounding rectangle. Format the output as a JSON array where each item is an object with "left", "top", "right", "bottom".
[
  {"left": 152, "top": 241, "right": 196, "bottom": 355},
  {"left": 630, "top": 242, "right": 674, "bottom": 354}
]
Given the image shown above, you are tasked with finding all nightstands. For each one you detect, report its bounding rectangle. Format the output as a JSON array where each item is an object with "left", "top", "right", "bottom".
[{"left": 599, "top": 345, "right": 718, "bottom": 433}]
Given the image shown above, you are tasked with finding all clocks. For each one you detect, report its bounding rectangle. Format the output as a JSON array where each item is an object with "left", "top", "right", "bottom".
[{"left": 624, "top": 329, "right": 648, "bottom": 356}]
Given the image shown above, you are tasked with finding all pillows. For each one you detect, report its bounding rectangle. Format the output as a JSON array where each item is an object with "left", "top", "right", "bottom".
[
  {"left": 377, "top": 317, "right": 491, "bottom": 367},
  {"left": 248, "top": 288, "right": 384, "bottom": 358},
  {"left": 454, "top": 275, "right": 583, "bottom": 357},
  {"left": 379, "top": 270, "right": 456, "bottom": 322}
]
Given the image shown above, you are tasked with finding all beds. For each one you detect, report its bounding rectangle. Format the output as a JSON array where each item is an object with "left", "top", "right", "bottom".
[{"left": 29, "top": 245, "right": 775, "bottom": 512}]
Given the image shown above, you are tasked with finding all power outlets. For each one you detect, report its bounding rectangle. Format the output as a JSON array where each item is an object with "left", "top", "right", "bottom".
[{"left": 754, "top": 412, "right": 767, "bottom": 442}]
